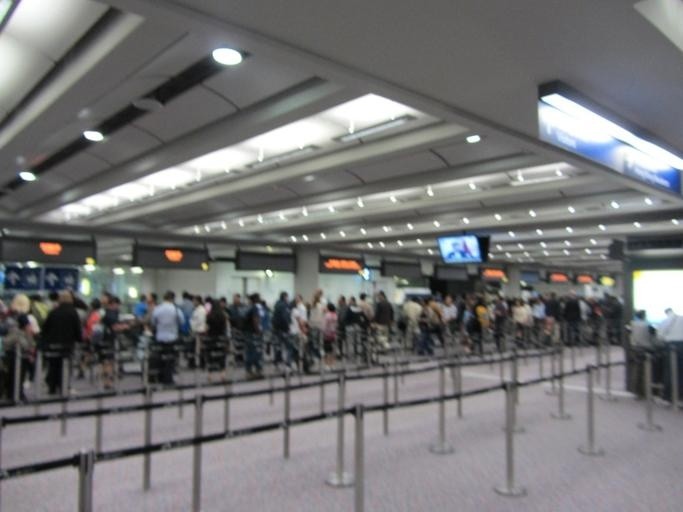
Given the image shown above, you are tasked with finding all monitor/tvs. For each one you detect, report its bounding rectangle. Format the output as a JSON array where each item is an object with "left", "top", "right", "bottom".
[{"left": 436, "top": 232, "right": 489, "bottom": 265}]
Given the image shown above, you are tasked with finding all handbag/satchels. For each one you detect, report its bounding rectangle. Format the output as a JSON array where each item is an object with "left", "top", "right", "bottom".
[{"left": 178, "top": 316, "right": 191, "bottom": 337}]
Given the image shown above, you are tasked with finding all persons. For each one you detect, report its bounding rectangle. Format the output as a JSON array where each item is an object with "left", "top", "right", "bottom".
[
  {"left": 625, "top": 309, "right": 656, "bottom": 397},
  {"left": 1, "top": 285, "right": 624, "bottom": 408},
  {"left": 656, "top": 307, "right": 683, "bottom": 409},
  {"left": 444, "top": 237, "right": 473, "bottom": 261}
]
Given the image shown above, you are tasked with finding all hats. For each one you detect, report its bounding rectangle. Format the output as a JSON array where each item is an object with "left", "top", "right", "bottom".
[{"left": 12, "top": 293, "right": 32, "bottom": 312}]
